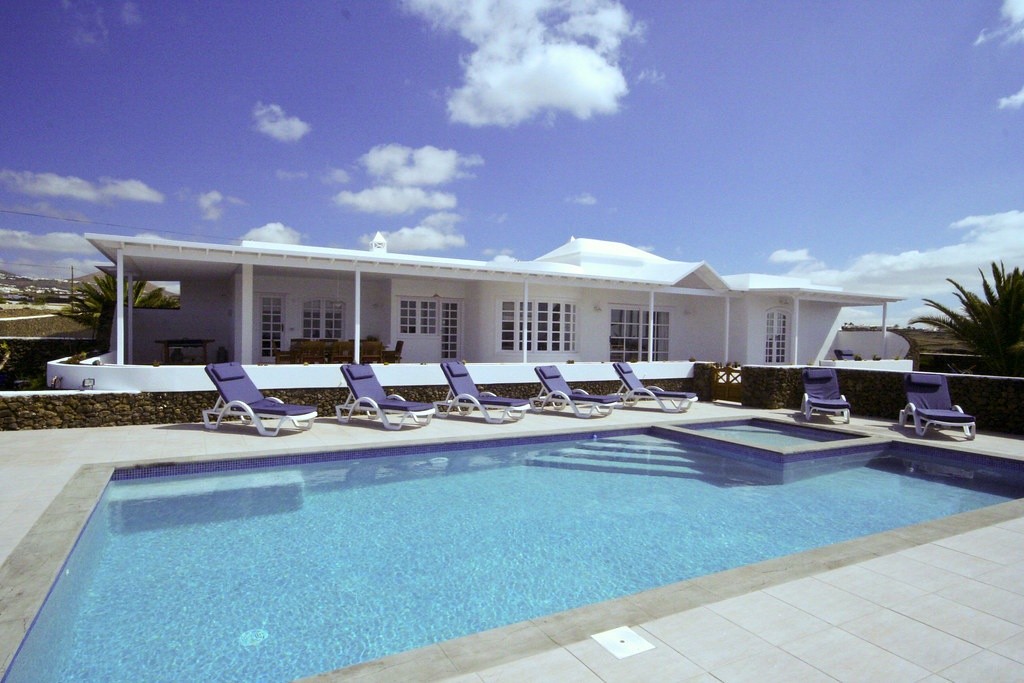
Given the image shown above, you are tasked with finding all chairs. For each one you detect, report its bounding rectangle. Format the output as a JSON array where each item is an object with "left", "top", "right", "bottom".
[
  {"left": 899, "top": 372, "right": 976, "bottom": 440},
  {"left": 607, "top": 362, "right": 699, "bottom": 413},
  {"left": 529, "top": 365, "right": 621, "bottom": 419},
  {"left": 201, "top": 360, "right": 318, "bottom": 436},
  {"left": 834, "top": 349, "right": 854, "bottom": 361},
  {"left": 800, "top": 367, "right": 851, "bottom": 424},
  {"left": 335, "top": 361, "right": 435, "bottom": 430},
  {"left": 433, "top": 360, "right": 532, "bottom": 424}
]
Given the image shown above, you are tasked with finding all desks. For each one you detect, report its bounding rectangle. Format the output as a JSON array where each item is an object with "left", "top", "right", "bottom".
[
  {"left": 270, "top": 337, "right": 404, "bottom": 364},
  {"left": 154, "top": 339, "right": 215, "bottom": 364}
]
